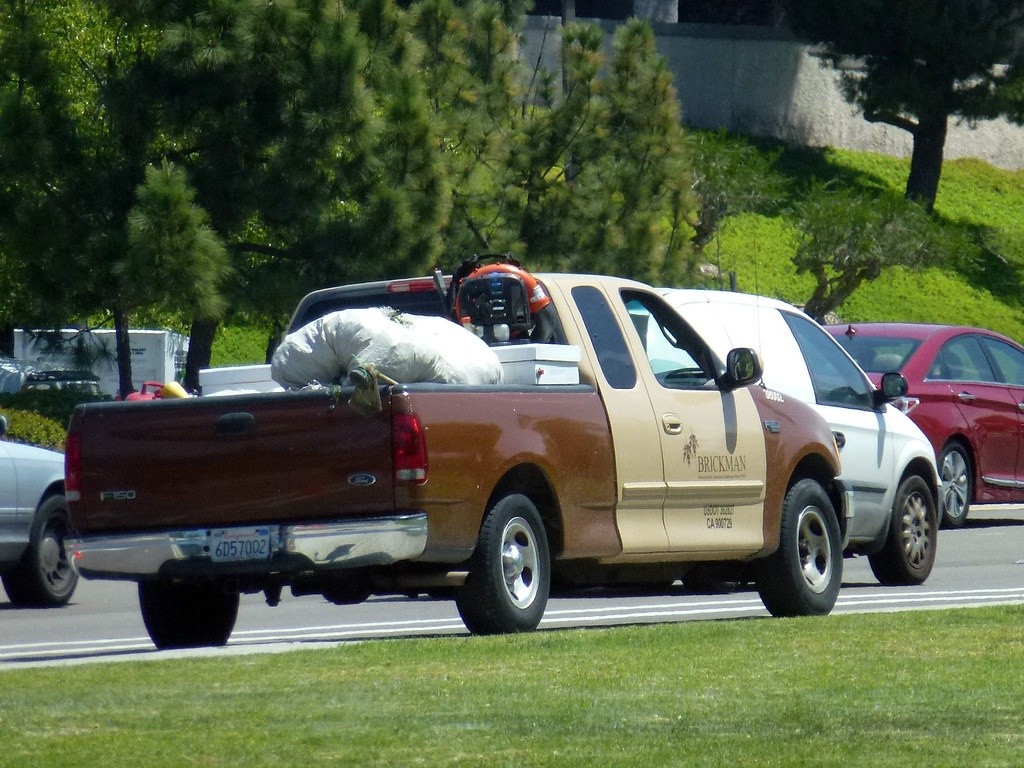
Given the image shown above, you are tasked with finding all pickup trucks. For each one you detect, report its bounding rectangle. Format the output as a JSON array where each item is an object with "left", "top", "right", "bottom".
[{"left": 63, "top": 272, "right": 855, "bottom": 651}]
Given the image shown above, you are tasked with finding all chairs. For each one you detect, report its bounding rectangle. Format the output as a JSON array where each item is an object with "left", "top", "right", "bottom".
[{"left": 871, "top": 353, "right": 906, "bottom": 374}]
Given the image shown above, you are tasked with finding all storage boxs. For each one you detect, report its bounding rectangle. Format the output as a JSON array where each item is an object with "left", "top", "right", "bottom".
[
  {"left": 199, "top": 363, "right": 287, "bottom": 396},
  {"left": 490, "top": 343, "right": 581, "bottom": 386}
]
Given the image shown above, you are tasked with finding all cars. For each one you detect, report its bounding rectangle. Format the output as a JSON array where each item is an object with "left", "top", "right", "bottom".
[
  {"left": 823, "top": 322, "right": 1024, "bottom": 531},
  {"left": 626, "top": 286, "right": 945, "bottom": 588},
  {"left": 0, "top": 415, "right": 80, "bottom": 610}
]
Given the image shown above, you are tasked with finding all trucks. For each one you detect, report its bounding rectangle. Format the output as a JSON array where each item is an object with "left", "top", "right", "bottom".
[{"left": 13, "top": 328, "right": 190, "bottom": 401}]
